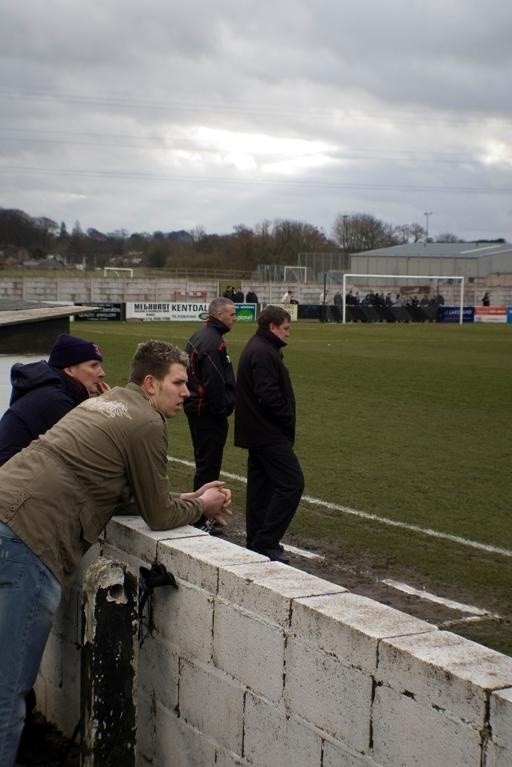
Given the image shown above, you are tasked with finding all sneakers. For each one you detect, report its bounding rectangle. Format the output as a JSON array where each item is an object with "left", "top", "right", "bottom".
[{"left": 192, "top": 516, "right": 225, "bottom": 536}]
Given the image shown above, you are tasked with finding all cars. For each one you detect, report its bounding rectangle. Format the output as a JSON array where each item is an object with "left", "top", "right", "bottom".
[{"left": 0, "top": 251, "right": 166, "bottom": 270}]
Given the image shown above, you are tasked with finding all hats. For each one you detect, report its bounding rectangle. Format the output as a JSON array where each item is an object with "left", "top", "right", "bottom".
[{"left": 48, "top": 333, "right": 103, "bottom": 369}]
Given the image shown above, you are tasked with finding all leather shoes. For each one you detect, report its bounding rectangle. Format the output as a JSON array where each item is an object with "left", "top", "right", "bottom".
[{"left": 246, "top": 539, "right": 290, "bottom": 563}]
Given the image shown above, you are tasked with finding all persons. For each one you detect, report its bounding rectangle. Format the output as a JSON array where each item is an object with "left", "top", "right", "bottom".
[
  {"left": 281, "top": 289, "right": 292, "bottom": 307},
  {"left": 234, "top": 304, "right": 306, "bottom": 562},
  {"left": 185, "top": 296, "right": 237, "bottom": 535},
  {"left": 319, "top": 288, "right": 328, "bottom": 322},
  {"left": 1, "top": 339, "right": 232, "bottom": 766},
  {"left": 0, "top": 332, "right": 113, "bottom": 462},
  {"left": 246, "top": 286, "right": 258, "bottom": 304},
  {"left": 481, "top": 292, "right": 490, "bottom": 306},
  {"left": 223, "top": 285, "right": 236, "bottom": 302},
  {"left": 236, "top": 286, "right": 244, "bottom": 302},
  {"left": 232, "top": 286, "right": 236, "bottom": 294},
  {"left": 334, "top": 289, "right": 445, "bottom": 322}
]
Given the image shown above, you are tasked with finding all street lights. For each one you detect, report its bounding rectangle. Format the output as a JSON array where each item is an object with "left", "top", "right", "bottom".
[
  {"left": 425, "top": 211, "right": 432, "bottom": 237},
  {"left": 343, "top": 214, "right": 347, "bottom": 252}
]
